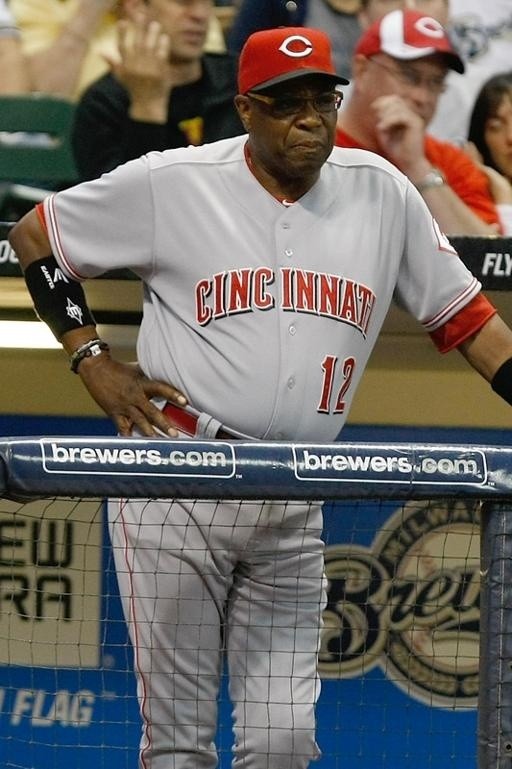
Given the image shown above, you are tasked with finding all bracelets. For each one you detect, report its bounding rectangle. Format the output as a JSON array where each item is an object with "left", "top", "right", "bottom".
[
  {"left": 491, "top": 358, "right": 512, "bottom": 407},
  {"left": 69, "top": 339, "right": 100, "bottom": 371},
  {"left": 71, "top": 343, "right": 109, "bottom": 374}
]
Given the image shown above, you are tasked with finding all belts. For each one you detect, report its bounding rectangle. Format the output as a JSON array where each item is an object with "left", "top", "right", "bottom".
[{"left": 162, "top": 403, "right": 246, "bottom": 442}]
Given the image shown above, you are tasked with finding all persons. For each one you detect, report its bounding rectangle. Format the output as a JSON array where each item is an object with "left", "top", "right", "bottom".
[
  {"left": 12, "top": 27, "right": 511, "bottom": 768},
  {"left": 0, "top": 0, "right": 512, "bottom": 235}
]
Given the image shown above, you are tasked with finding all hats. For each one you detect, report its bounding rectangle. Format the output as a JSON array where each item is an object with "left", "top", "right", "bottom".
[
  {"left": 354, "top": 9, "right": 464, "bottom": 74},
  {"left": 237, "top": 26, "right": 350, "bottom": 96}
]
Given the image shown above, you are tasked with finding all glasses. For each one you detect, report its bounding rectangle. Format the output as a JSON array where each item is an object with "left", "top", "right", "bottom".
[{"left": 247, "top": 91, "right": 343, "bottom": 114}]
